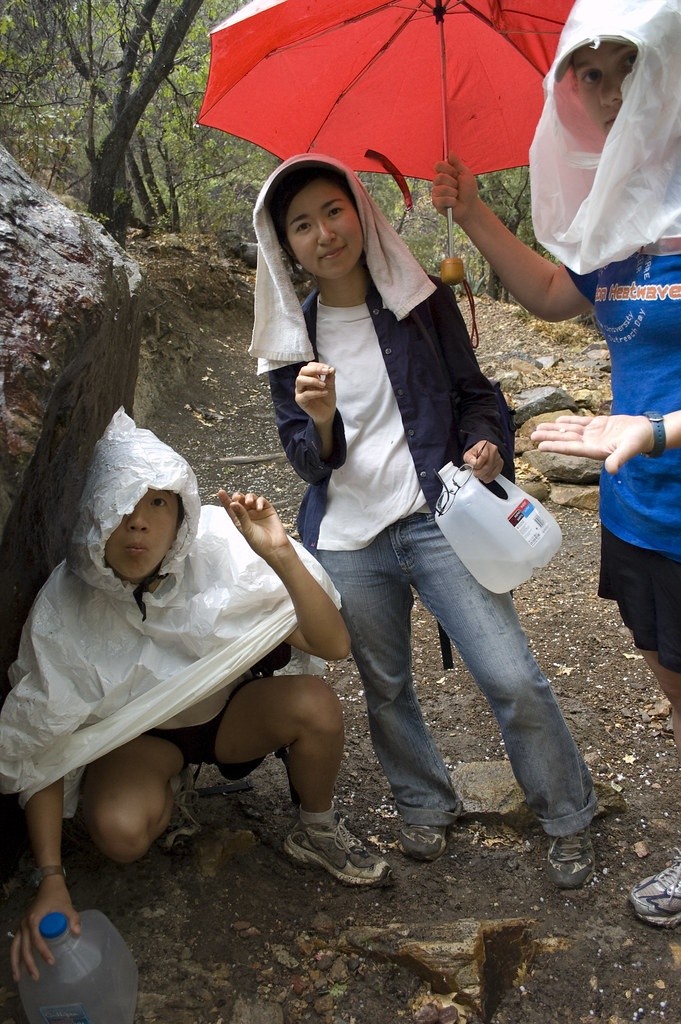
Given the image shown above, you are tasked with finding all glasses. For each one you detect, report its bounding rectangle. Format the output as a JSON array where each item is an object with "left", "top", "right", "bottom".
[{"left": 433, "top": 430, "right": 489, "bottom": 515}]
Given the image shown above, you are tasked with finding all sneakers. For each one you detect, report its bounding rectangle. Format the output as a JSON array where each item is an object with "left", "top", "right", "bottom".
[
  {"left": 628, "top": 847, "right": 681, "bottom": 928},
  {"left": 548, "top": 825, "right": 595, "bottom": 888},
  {"left": 399, "top": 824, "right": 447, "bottom": 862},
  {"left": 283, "top": 810, "right": 392, "bottom": 887},
  {"left": 154, "top": 766, "right": 202, "bottom": 850}
]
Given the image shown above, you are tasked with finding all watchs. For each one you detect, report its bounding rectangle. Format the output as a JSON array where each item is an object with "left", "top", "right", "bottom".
[{"left": 32, "top": 865, "right": 66, "bottom": 891}]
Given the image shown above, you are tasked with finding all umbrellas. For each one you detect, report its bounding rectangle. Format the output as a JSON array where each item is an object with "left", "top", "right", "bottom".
[{"left": 193, "top": 1, "right": 577, "bottom": 284}]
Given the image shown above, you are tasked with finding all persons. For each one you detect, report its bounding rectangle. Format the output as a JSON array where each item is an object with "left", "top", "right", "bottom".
[
  {"left": 434, "top": 1, "right": 681, "bottom": 925},
  {"left": 247, "top": 155, "right": 599, "bottom": 886},
  {"left": 0, "top": 407, "right": 394, "bottom": 982}
]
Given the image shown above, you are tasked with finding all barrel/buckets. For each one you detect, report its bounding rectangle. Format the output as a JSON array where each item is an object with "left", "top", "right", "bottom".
[
  {"left": 17, "top": 909, "right": 139, "bottom": 1024},
  {"left": 433, "top": 460, "right": 561, "bottom": 594}
]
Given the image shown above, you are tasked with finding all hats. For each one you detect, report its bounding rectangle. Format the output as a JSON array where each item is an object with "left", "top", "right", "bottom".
[
  {"left": 553, "top": 35, "right": 637, "bottom": 83},
  {"left": 264, "top": 158, "right": 348, "bottom": 225}
]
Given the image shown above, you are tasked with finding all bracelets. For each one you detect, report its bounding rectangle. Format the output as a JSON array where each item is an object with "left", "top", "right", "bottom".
[{"left": 640, "top": 412, "right": 666, "bottom": 458}]
